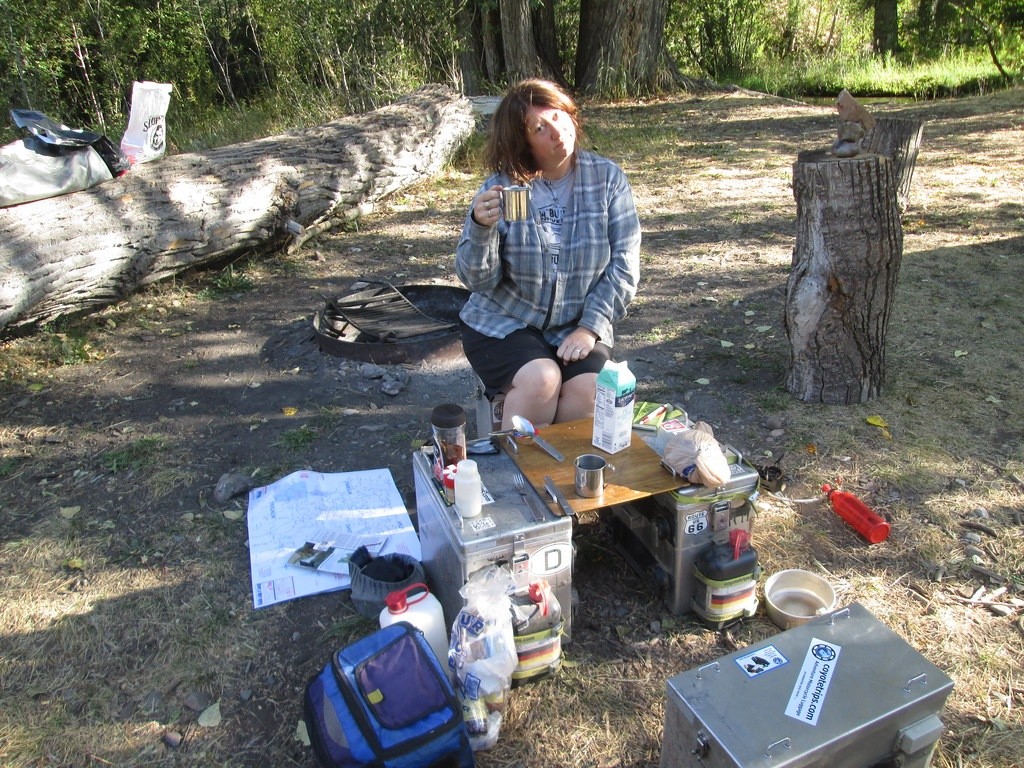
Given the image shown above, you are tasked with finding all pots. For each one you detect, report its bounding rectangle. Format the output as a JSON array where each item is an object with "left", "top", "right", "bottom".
[{"left": 764, "top": 569, "right": 836, "bottom": 630}]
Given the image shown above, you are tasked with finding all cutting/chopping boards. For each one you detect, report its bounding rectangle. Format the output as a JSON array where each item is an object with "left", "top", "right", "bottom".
[{"left": 502, "top": 416, "right": 690, "bottom": 517}]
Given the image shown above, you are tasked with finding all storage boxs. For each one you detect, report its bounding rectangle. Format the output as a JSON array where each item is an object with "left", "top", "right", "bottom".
[
  {"left": 660, "top": 601, "right": 955, "bottom": 768},
  {"left": 599, "top": 444, "right": 759, "bottom": 617},
  {"left": 412, "top": 451, "right": 572, "bottom": 646}
]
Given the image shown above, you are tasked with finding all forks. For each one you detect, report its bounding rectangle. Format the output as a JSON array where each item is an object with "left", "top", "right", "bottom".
[{"left": 513, "top": 474, "right": 546, "bottom": 521}]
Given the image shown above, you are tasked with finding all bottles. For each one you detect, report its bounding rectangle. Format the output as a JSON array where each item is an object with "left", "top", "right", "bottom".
[
  {"left": 453, "top": 459, "right": 482, "bottom": 517},
  {"left": 508, "top": 581, "right": 564, "bottom": 687},
  {"left": 689, "top": 529, "right": 759, "bottom": 631},
  {"left": 442, "top": 463, "right": 457, "bottom": 503},
  {"left": 432, "top": 403, "right": 467, "bottom": 485},
  {"left": 378, "top": 583, "right": 449, "bottom": 678},
  {"left": 821, "top": 484, "right": 891, "bottom": 544}
]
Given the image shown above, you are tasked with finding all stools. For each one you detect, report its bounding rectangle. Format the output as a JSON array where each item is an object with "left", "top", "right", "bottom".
[{"left": 474, "top": 373, "right": 505, "bottom": 438}]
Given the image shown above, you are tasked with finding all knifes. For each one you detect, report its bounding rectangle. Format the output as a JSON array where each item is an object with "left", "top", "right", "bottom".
[{"left": 544, "top": 476, "right": 575, "bottom": 516}]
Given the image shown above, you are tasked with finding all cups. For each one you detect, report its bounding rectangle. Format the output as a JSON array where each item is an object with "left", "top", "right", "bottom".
[
  {"left": 497, "top": 186, "right": 530, "bottom": 222},
  {"left": 574, "top": 454, "right": 616, "bottom": 498}
]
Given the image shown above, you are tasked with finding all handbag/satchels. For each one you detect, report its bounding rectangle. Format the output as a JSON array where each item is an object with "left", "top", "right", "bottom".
[
  {"left": 9, "top": 107, "right": 125, "bottom": 177},
  {"left": 302, "top": 621, "right": 474, "bottom": 768},
  {"left": 659, "top": 428, "right": 731, "bottom": 488},
  {"left": 446, "top": 563, "right": 518, "bottom": 751},
  {"left": 121, "top": 80, "right": 172, "bottom": 168}
]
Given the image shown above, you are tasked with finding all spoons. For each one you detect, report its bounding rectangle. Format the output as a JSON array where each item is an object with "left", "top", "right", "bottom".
[{"left": 512, "top": 415, "right": 565, "bottom": 461}]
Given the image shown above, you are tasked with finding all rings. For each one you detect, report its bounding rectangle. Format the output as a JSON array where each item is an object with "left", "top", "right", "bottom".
[
  {"left": 574, "top": 350, "right": 581, "bottom": 353},
  {"left": 484, "top": 200, "right": 492, "bottom": 209},
  {"left": 488, "top": 210, "right": 491, "bottom": 218}
]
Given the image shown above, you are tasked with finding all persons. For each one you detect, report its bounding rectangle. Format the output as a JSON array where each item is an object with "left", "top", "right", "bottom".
[{"left": 454, "top": 78, "right": 642, "bottom": 431}]
